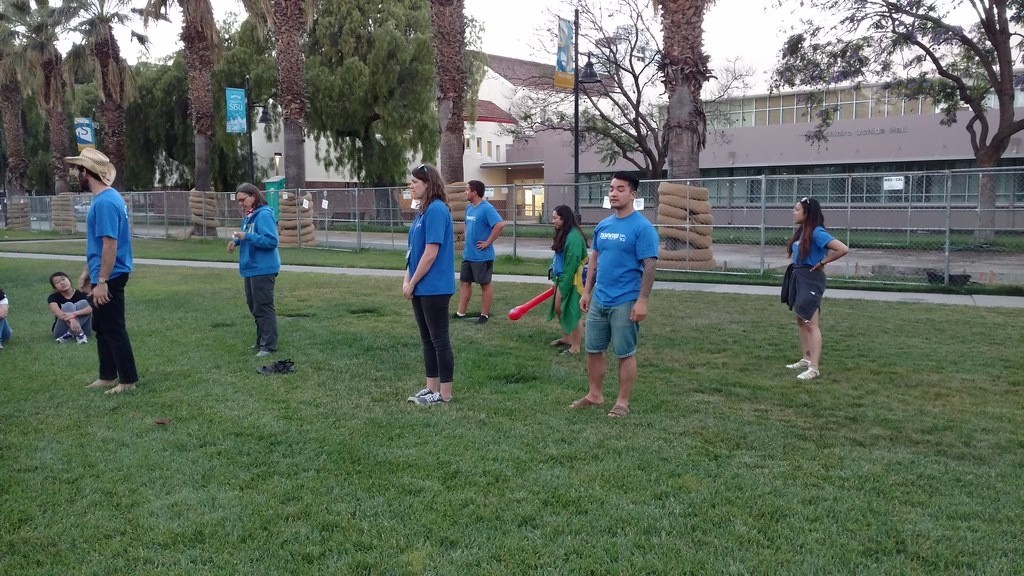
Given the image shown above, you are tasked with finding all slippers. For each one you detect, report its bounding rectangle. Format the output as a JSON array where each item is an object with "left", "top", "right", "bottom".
[
  {"left": 560, "top": 350, "right": 576, "bottom": 355},
  {"left": 550, "top": 340, "right": 567, "bottom": 346},
  {"left": 570, "top": 398, "right": 604, "bottom": 408},
  {"left": 608, "top": 405, "right": 629, "bottom": 418}
]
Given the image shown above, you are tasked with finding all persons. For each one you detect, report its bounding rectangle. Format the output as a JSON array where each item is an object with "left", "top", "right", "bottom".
[
  {"left": 546, "top": 205, "right": 589, "bottom": 354},
  {"left": 448, "top": 181, "right": 505, "bottom": 325},
  {"left": 63, "top": 148, "right": 138, "bottom": 394},
  {"left": 570, "top": 171, "right": 659, "bottom": 418},
  {"left": 781, "top": 197, "right": 849, "bottom": 379},
  {"left": 0, "top": 289, "right": 13, "bottom": 348},
  {"left": 47, "top": 272, "right": 93, "bottom": 344},
  {"left": 226, "top": 183, "right": 281, "bottom": 357},
  {"left": 401, "top": 165, "right": 455, "bottom": 406}
]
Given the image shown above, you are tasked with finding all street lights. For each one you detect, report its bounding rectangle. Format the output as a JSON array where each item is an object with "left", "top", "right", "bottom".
[
  {"left": 571, "top": 9, "right": 605, "bottom": 226},
  {"left": 243, "top": 73, "right": 272, "bottom": 186}
]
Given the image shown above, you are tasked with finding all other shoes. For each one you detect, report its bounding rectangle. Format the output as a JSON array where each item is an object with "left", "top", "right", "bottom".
[
  {"left": 250, "top": 345, "right": 259, "bottom": 350},
  {"left": 450, "top": 313, "right": 465, "bottom": 318},
  {"left": 256, "top": 352, "right": 272, "bottom": 359},
  {"left": 477, "top": 315, "right": 488, "bottom": 325}
]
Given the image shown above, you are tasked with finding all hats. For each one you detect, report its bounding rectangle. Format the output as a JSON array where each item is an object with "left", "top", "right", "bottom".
[{"left": 64, "top": 148, "right": 116, "bottom": 185}]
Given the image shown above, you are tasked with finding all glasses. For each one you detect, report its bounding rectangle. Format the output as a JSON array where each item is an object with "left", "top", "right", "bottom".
[
  {"left": 237, "top": 195, "right": 248, "bottom": 202},
  {"left": 801, "top": 197, "right": 810, "bottom": 203},
  {"left": 418, "top": 164, "right": 428, "bottom": 176}
]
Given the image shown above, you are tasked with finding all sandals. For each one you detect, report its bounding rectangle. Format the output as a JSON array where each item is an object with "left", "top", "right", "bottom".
[
  {"left": 797, "top": 368, "right": 820, "bottom": 380},
  {"left": 786, "top": 359, "right": 812, "bottom": 369},
  {"left": 257, "top": 359, "right": 294, "bottom": 374}
]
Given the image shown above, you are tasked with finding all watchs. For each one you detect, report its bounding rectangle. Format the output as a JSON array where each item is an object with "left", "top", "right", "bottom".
[{"left": 99, "top": 278, "right": 108, "bottom": 283}]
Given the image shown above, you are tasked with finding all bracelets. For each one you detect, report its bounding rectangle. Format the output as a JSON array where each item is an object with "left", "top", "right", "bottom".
[{"left": 821, "top": 261, "right": 824, "bottom": 265}]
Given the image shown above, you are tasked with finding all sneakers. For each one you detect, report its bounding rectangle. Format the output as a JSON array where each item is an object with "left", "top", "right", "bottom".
[
  {"left": 56, "top": 332, "right": 74, "bottom": 343},
  {"left": 76, "top": 333, "right": 88, "bottom": 344},
  {"left": 407, "top": 389, "right": 433, "bottom": 402},
  {"left": 416, "top": 392, "right": 453, "bottom": 405}
]
